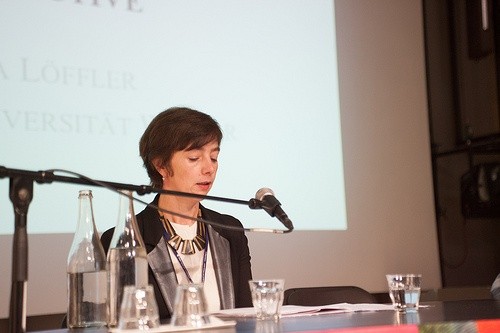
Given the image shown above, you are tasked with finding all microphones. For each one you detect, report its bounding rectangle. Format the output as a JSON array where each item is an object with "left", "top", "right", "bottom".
[{"left": 255, "top": 187, "right": 294, "bottom": 230}]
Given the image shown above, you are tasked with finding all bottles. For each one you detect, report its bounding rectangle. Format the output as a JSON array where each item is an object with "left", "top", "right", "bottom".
[
  {"left": 107, "top": 190, "right": 148, "bottom": 328},
  {"left": 67, "top": 190, "right": 111, "bottom": 333}
]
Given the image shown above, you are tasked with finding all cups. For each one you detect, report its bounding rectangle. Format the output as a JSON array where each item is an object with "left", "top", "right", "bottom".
[
  {"left": 169, "top": 284, "right": 209, "bottom": 328},
  {"left": 249, "top": 280, "right": 284, "bottom": 320},
  {"left": 386, "top": 273, "right": 421, "bottom": 313},
  {"left": 116, "top": 285, "right": 160, "bottom": 331}
]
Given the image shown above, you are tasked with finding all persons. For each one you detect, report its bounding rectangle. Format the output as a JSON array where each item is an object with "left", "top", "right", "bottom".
[{"left": 61, "top": 107, "right": 254, "bottom": 329}]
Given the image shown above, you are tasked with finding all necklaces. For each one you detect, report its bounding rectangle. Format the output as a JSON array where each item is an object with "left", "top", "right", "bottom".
[
  {"left": 157, "top": 209, "right": 206, "bottom": 255},
  {"left": 162, "top": 225, "right": 209, "bottom": 284}
]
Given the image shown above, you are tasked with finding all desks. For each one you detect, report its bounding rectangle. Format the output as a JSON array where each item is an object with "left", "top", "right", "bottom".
[{"left": 36, "top": 299, "right": 500, "bottom": 333}]
